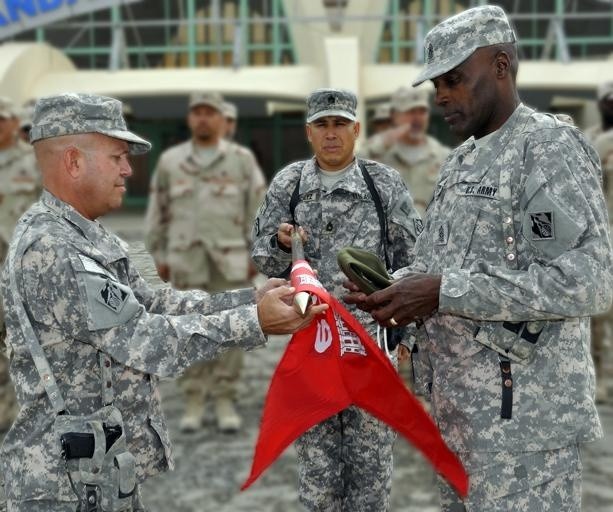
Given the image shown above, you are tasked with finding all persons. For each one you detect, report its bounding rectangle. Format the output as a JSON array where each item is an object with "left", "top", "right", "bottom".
[
  {"left": 582, "top": 91, "right": 612, "bottom": 405},
  {"left": 142, "top": 92, "right": 263, "bottom": 431},
  {"left": 357, "top": 90, "right": 452, "bottom": 415},
  {"left": 342, "top": 3, "right": 613, "bottom": 512},
  {"left": 1, "top": 98, "right": 41, "bottom": 269},
  {"left": 0, "top": 94, "right": 331, "bottom": 512},
  {"left": 247, "top": 88, "right": 424, "bottom": 512}
]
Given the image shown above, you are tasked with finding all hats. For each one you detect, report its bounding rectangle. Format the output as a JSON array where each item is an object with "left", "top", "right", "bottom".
[
  {"left": 305, "top": 91, "right": 358, "bottom": 123},
  {"left": 0, "top": 93, "right": 153, "bottom": 157},
  {"left": 188, "top": 89, "right": 239, "bottom": 121},
  {"left": 373, "top": 86, "right": 431, "bottom": 122},
  {"left": 412, "top": 6, "right": 518, "bottom": 89},
  {"left": 596, "top": 81, "right": 612, "bottom": 103}
]
